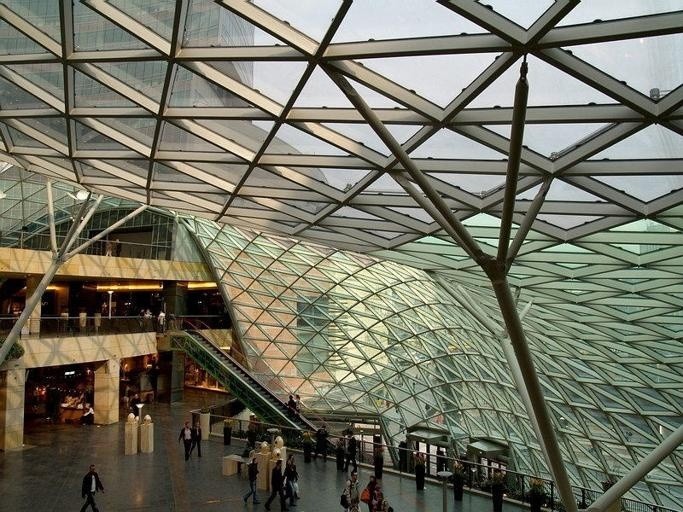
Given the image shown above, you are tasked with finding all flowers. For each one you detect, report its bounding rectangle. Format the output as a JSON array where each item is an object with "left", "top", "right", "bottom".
[{"left": 301, "top": 430, "right": 311, "bottom": 442}]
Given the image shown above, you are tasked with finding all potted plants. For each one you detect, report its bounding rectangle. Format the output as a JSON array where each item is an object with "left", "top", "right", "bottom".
[
  {"left": 223, "top": 418, "right": 234, "bottom": 446},
  {"left": 199, "top": 390, "right": 211, "bottom": 441}
]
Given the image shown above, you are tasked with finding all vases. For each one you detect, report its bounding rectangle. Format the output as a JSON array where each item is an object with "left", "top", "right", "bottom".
[{"left": 299, "top": 441, "right": 545, "bottom": 512}]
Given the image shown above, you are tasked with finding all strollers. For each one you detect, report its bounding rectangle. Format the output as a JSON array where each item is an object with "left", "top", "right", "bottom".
[{"left": 379, "top": 498, "right": 393, "bottom": 512}]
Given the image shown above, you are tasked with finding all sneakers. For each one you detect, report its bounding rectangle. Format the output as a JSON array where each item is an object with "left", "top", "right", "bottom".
[
  {"left": 295, "top": 497, "right": 301, "bottom": 500},
  {"left": 264, "top": 503, "right": 271, "bottom": 512},
  {"left": 288, "top": 504, "right": 297, "bottom": 507},
  {"left": 243, "top": 495, "right": 248, "bottom": 504},
  {"left": 198, "top": 454, "right": 202, "bottom": 457},
  {"left": 185, "top": 454, "right": 189, "bottom": 460},
  {"left": 280, "top": 507, "right": 290, "bottom": 512},
  {"left": 252, "top": 500, "right": 261, "bottom": 505},
  {"left": 189, "top": 451, "right": 192, "bottom": 457}
]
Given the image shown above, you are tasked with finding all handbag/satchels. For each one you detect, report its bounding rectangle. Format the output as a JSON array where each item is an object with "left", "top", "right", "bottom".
[
  {"left": 360, "top": 487, "right": 371, "bottom": 504},
  {"left": 340, "top": 494, "right": 350, "bottom": 510}
]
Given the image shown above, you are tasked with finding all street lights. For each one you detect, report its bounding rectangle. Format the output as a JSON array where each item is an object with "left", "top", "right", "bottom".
[
  {"left": 107, "top": 290, "right": 114, "bottom": 319},
  {"left": 434, "top": 470, "right": 453, "bottom": 512},
  {"left": 134, "top": 402, "right": 146, "bottom": 453}
]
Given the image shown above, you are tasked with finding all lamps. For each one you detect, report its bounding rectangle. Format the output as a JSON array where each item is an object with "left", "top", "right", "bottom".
[{"left": 135, "top": 403, "right": 146, "bottom": 423}]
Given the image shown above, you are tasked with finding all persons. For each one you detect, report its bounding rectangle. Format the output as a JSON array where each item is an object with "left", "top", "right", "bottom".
[
  {"left": 25, "top": 370, "right": 154, "bottom": 428},
  {"left": 41, "top": 305, "right": 176, "bottom": 340},
  {"left": 79, "top": 464, "right": 104, "bottom": 512},
  {"left": 105, "top": 237, "right": 114, "bottom": 257},
  {"left": 294, "top": 394, "right": 301, "bottom": 414},
  {"left": 284, "top": 395, "right": 296, "bottom": 415},
  {"left": 175, "top": 415, "right": 393, "bottom": 512},
  {"left": 114, "top": 237, "right": 121, "bottom": 257}
]
